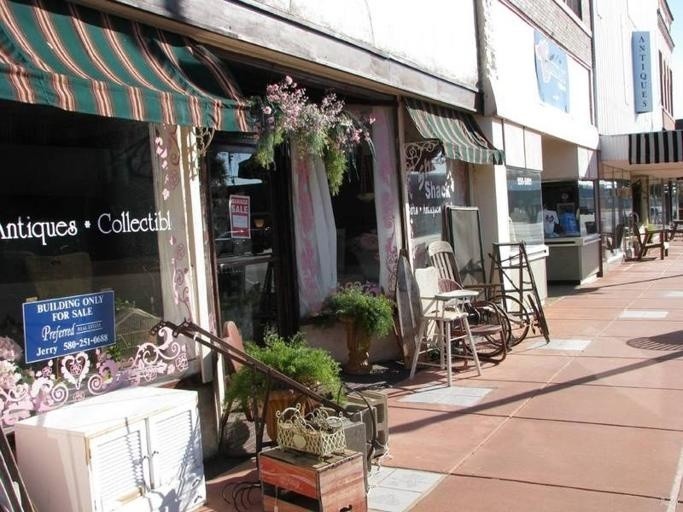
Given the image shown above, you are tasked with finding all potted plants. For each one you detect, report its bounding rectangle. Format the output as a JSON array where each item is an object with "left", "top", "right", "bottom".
[
  {"left": 312, "top": 281, "right": 398, "bottom": 374},
  {"left": 221, "top": 323, "right": 349, "bottom": 443}
]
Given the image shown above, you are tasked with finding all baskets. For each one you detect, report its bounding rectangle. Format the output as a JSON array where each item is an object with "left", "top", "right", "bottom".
[{"left": 274, "top": 403, "right": 346, "bottom": 461}]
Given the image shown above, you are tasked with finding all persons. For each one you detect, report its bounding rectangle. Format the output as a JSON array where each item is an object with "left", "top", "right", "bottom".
[
  {"left": 536, "top": 201, "right": 559, "bottom": 238},
  {"left": 510, "top": 199, "right": 531, "bottom": 223}
]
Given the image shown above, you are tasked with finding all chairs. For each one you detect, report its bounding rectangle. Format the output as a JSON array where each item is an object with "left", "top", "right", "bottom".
[
  {"left": 406, "top": 241, "right": 530, "bottom": 387},
  {"left": 624, "top": 210, "right": 682, "bottom": 262}
]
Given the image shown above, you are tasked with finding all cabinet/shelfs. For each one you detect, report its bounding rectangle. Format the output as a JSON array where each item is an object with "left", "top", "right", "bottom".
[
  {"left": 11, "top": 384, "right": 209, "bottom": 512},
  {"left": 258, "top": 443, "right": 368, "bottom": 512}
]
